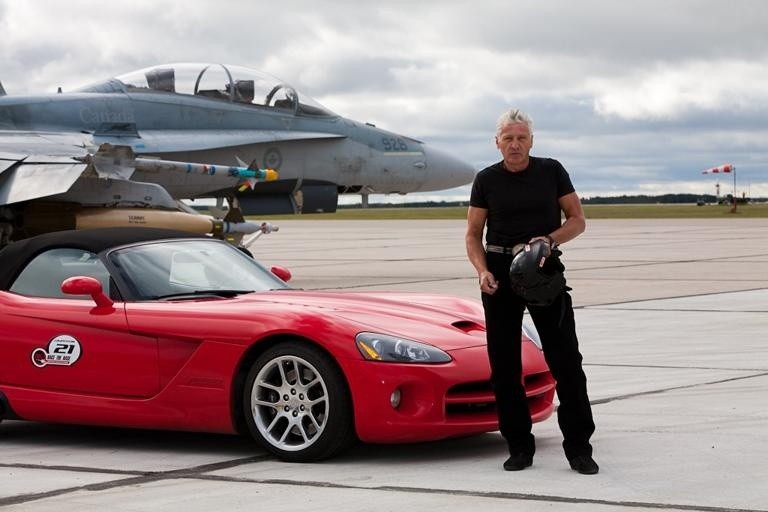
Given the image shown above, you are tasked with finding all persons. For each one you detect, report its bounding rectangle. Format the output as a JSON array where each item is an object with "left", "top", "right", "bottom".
[{"left": 465, "top": 109, "right": 599, "bottom": 475}]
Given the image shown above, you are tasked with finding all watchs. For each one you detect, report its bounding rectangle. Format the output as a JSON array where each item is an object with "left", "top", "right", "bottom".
[{"left": 545, "top": 235, "right": 555, "bottom": 250}]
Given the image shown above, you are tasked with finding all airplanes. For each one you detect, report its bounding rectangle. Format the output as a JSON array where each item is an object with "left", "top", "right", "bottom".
[{"left": 0, "top": 61, "right": 476, "bottom": 249}]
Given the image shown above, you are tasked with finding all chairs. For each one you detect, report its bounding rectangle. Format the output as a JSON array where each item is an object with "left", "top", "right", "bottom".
[{"left": 140, "top": 252, "right": 173, "bottom": 290}]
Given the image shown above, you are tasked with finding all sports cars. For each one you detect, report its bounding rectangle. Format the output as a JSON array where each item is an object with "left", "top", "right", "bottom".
[{"left": 0, "top": 227, "right": 558, "bottom": 463}]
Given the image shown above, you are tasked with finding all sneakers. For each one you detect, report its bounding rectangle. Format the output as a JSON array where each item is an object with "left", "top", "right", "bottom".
[
  {"left": 562, "top": 440, "right": 599, "bottom": 475},
  {"left": 503, "top": 434, "right": 535, "bottom": 470}
]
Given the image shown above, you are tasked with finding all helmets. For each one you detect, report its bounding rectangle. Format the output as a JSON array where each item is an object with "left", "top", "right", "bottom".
[{"left": 509, "top": 239, "right": 572, "bottom": 307}]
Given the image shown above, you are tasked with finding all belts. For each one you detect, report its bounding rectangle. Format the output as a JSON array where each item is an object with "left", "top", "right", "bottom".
[{"left": 483, "top": 243, "right": 513, "bottom": 256}]
